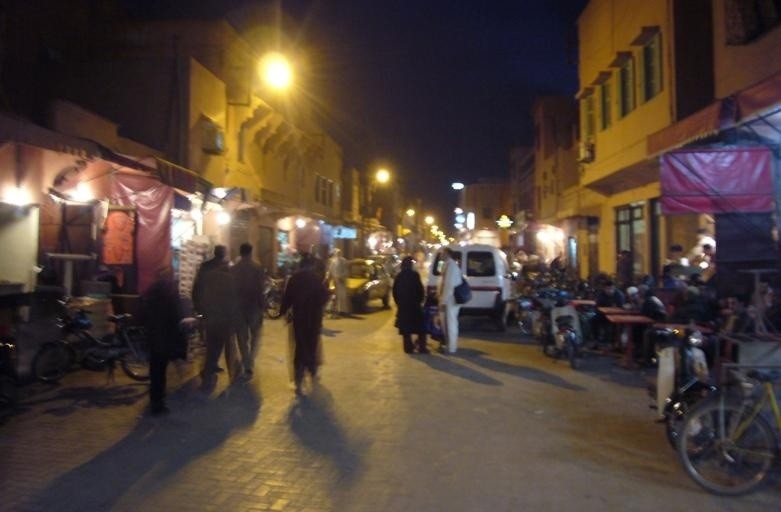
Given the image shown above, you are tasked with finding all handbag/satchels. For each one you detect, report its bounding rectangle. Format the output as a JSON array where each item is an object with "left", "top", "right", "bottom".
[{"left": 454, "top": 279, "right": 472, "bottom": 303}]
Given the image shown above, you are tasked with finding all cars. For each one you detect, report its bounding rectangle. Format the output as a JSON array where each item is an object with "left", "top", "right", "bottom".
[{"left": 330, "top": 259, "right": 390, "bottom": 311}]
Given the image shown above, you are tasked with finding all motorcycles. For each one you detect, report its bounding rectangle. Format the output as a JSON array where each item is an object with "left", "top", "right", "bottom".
[{"left": 518, "top": 279, "right": 607, "bottom": 369}]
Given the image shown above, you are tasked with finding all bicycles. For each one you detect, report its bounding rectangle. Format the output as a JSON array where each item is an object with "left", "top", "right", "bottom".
[
  {"left": 677, "top": 367, "right": 781, "bottom": 497},
  {"left": 263, "top": 274, "right": 284, "bottom": 318},
  {"left": 34, "top": 298, "right": 151, "bottom": 382}
]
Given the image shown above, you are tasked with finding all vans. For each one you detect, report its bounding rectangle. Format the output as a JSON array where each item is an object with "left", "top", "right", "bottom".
[{"left": 428, "top": 243, "right": 519, "bottom": 330}]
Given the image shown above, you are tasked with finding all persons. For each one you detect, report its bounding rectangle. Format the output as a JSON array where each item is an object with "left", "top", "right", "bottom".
[
  {"left": 271, "top": 241, "right": 356, "bottom": 334},
  {"left": 277, "top": 251, "right": 328, "bottom": 396},
  {"left": 392, "top": 255, "right": 432, "bottom": 355},
  {"left": 232, "top": 241, "right": 268, "bottom": 382},
  {"left": 436, "top": 247, "right": 467, "bottom": 357},
  {"left": 191, "top": 244, "right": 233, "bottom": 380},
  {"left": 587, "top": 225, "right": 781, "bottom": 373},
  {"left": 135, "top": 263, "right": 188, "bottom": 416}
]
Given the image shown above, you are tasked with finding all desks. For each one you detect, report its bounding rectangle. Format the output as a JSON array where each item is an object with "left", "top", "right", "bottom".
[{"left": 569, "top": 299, "right": 713, "bottom": 371}]
